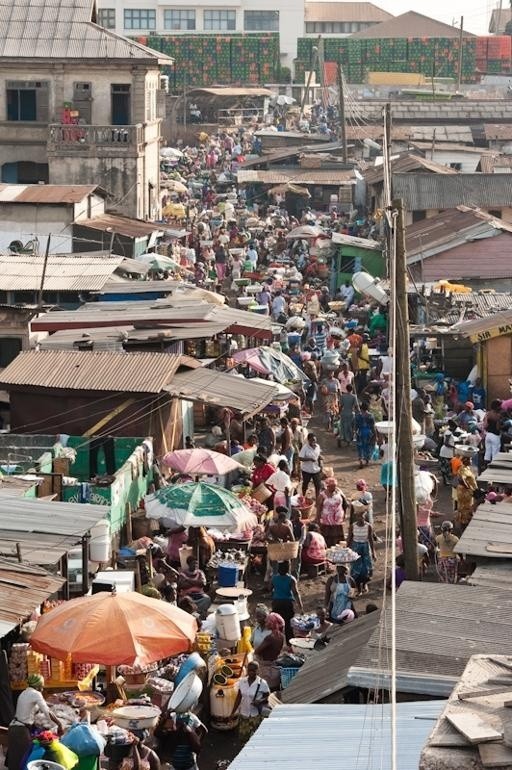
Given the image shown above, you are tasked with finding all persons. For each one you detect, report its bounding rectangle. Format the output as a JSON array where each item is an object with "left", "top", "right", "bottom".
[{"left": 4, "top": 93, "right": 511, "bottom": 768}]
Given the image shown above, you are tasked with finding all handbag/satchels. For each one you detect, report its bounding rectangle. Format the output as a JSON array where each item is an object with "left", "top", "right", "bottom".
[
  {"left": 372, "top": 444, "right": 380, "bottom": 460},
  {"left": 259, "top": 704, "right": 272, "bottom": 717}
]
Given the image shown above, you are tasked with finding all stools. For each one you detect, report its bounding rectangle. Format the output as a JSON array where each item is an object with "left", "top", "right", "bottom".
[{"left": 308, "top": 562, "right": 327, "bottom": 577}]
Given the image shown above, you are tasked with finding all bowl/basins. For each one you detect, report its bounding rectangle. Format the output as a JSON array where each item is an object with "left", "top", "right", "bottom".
[
  {"left": 454, "top": 443, "right": 479, "bottom": 458},
  {"left": 289, "top": 637, "right": 328, "bottom": 656},
  {"left": 167, "top": 651, "right": 210, "bottom": 717},
  {"left": 412, "top": 434, "right": 427, "bottom": 450},
  {"left": 198, "top": 209, "right": 360, "bottom": 371},
  {"left": 374, "top": 420, "right": 394, "bottom": 433},
  {"left": 25, "top": 759, "right": 68, "bottom": 770},
  {"left": 112, "top": 704, "right": 161, "bottom": 731}
]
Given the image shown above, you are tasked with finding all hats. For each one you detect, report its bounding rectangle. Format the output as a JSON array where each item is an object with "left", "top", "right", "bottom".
[{"left": 28, "top": 675, "right": 43, "bottom": 686}]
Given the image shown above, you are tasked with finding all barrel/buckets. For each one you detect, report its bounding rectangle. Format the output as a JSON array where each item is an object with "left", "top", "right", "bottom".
[
  {"left": 209, "top": 603, "right": 247, "bottom": 730},
  {"left": 90, "top": 540, "right": 111, "bottom": 563},
  {"left": 91, "top": 519, "right": 110, "bottom": 540}
]
